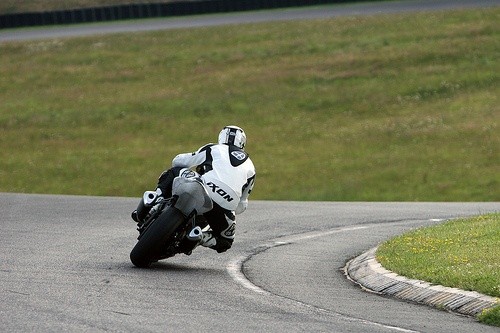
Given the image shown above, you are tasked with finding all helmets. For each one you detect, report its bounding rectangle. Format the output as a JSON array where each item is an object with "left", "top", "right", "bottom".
[{"left": 218, "top": 124, "right": 246, "bottom": 149}]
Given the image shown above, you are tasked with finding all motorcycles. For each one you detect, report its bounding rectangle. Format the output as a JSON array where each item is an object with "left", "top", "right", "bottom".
[{"left": 130, "top": 174, "right": 215, "bottom": 269}]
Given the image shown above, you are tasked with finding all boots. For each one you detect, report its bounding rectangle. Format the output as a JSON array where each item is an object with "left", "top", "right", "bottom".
[
  {"left": 147, "top": 188, "right": 164, "bottom": 214},
  {"left": 194, "top": 226, "right": 217, "bottom": 249}
]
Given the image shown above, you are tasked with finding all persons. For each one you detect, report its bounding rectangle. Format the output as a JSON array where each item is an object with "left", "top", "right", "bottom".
[{"left": 149, "top": 126, "right": 255, "bottom": 253}]
